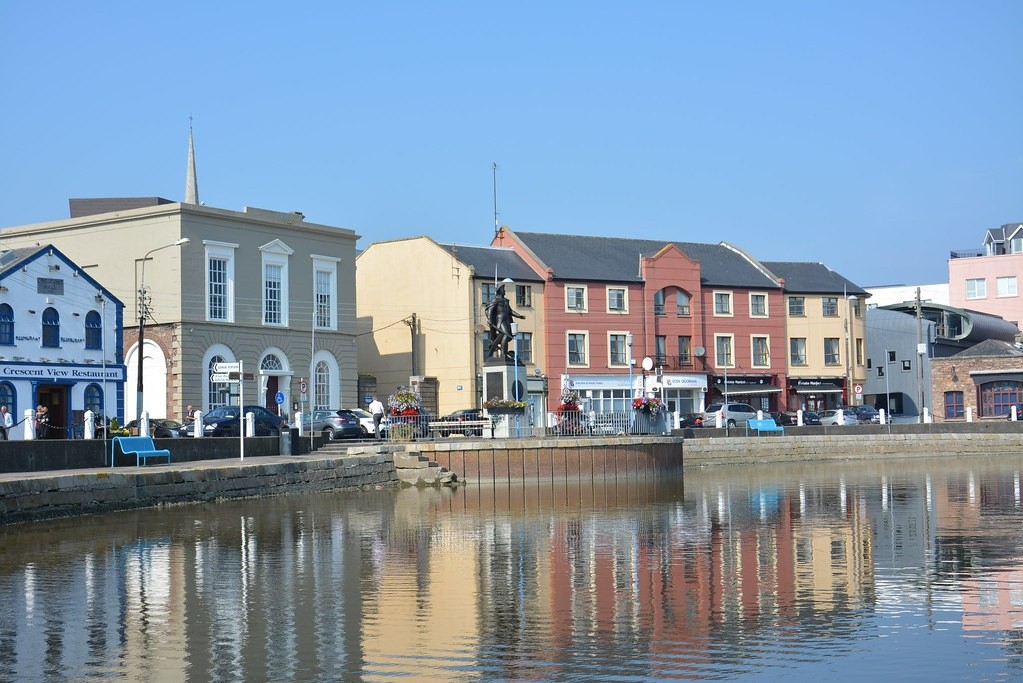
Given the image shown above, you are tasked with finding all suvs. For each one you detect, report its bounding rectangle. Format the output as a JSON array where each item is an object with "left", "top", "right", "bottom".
[
  {"left": 703, "top": 402, "right": 770, "bottom": 427},
  {"left": 819, "top": 408, "right": 857, "bottom": 426},
  {"left": 356, "top": 410, "right": 393, "bottom": 437},
  {"left": 290, "top": 409, "right": 360, "bottom": 439},
  {"left": 382, "top": 407, "right": 431, "bottom": 438}
]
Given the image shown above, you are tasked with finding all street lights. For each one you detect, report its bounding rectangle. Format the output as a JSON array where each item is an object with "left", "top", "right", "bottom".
[
  {"left": 311, "top": 311, "right": 318, "bottom": 452},
  {"left": 135, "top": 238, "right": 191, "bottom": 436},
  {"left": 845, "top": 295, "right": 859, "bottom": 406},
  {"left": 884, "top": 347, "right": 895, "bottom": 435},
  {"left": 626, "top": 334, "right": 635, "bottom": 427},
  {"left": 511, "top": 323, "right": 521, "bottom": 438},
  {"left": 723, "top": 341, "right": 730, "bottom": 437}
]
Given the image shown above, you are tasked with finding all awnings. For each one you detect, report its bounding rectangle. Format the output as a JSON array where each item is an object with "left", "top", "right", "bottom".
[
  {"left": 793, "top": 384, "right": 843, "bottom": 395},
  {"left": 713, "top": 384, "right": 783, "bottom": 397}
]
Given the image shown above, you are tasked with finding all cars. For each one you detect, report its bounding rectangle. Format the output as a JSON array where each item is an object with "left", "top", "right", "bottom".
[
  {"left": 1003, "top": 402, "right": 1023, "bottom": 420},
  {"left": 125, "top": 418, "right": 188, "bottom": 437},
  {"left": 843, "top": 405, "right": 892, "bottom": 424},
  {"left": 770, "top": 411, "right": 797, "bottom": 426},
  {"left": 560, "top": 412, "right": 629, "bottom": 436},
  {"left": 803, "top": 411, "right": 822, "bottom": 425},
  {"left": 439, "top": 408, "right": 489, "bottom": 438},
  {"left": 680, "top": 413, "right": 703, "bottom": 428},
  {"left": 180, "top": 404, "right": 286, "bottom": 438}
]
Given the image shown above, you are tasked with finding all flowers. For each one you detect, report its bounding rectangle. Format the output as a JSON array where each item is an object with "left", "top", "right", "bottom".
[
  {"left": 384, "top": 383, "right": 423, "bottom": 416},
  {"left": 481, "top": 395, "right": 529, "bottom": 409},
  {"left": 631, "top": 397, "right": 669, "bottom": 423},
  {"left": 557, "top": 392, "right": 582, "bottom": 413}
]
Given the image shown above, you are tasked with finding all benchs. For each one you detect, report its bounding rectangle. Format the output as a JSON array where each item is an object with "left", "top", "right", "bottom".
[
  {"left": 111, "top": 435, "right": 170, "bottom": 468},
  {"left": 745, "top": 418, "right": 785, "bottom": 438},
  {"left": 132, "top": 427, "right": 156, "bottom": 438}
]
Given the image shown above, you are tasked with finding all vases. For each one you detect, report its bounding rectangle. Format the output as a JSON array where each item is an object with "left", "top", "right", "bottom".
[{"left": 488, "top": 406, "right": 524, "bottom": 415}]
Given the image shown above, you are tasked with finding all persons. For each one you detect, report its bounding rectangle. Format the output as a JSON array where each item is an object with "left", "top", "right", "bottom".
[
  {"left": 97, "top": 410, "right": 110, "bottom": 439},
  {"left": 369, "top": 396, "right": 386, "bottom": 442},
  {"left": 486, "top": 283, "right": 526, "bottom": 358},
  {"left": 1, "top": 406, "right": 13, "bottom": 439},
  {"left": 35, "top": 405, "right": 49, "bottom": 439},
  {"left": 187, "top": 405, "right": 194, "bottom": 422}
]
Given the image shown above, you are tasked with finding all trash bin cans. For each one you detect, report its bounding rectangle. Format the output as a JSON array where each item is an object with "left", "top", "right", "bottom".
[{"left": 279, "top": 424, "right": 291, "bottom": 455}]
